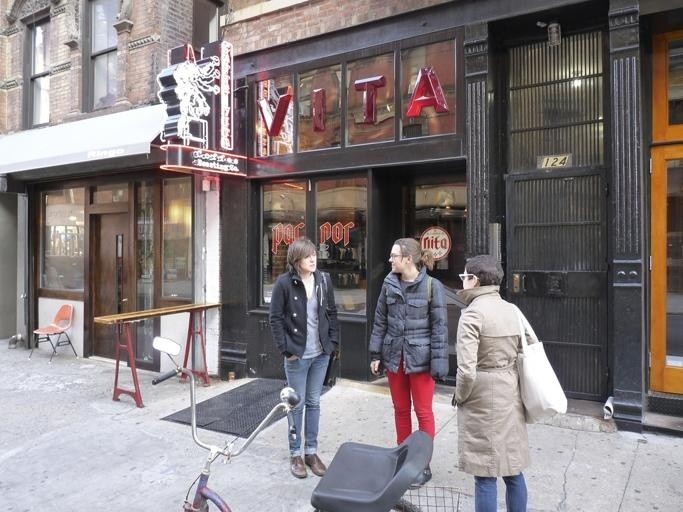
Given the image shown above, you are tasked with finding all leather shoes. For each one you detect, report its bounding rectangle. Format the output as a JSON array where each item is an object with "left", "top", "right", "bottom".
[
  {"left": 407, "top": 463, "right": 432, "bottom": 490},
  {"left": 290, "top": 456, "right": 308, "bottom": 479},
  {"left": 305, "top": 453, "right": 326, "bottom": 476}
]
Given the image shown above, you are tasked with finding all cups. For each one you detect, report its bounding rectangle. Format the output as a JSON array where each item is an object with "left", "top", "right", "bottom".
[{"left": 320, "top": 243, "right": 330, "bottom": 259}]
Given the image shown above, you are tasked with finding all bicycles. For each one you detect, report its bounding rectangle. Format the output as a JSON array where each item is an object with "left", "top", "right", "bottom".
[{"left": 152, "top": 337, "right": 433, "bottom": 512}]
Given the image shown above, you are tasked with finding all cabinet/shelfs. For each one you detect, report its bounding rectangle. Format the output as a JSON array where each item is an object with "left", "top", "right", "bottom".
[{"left": 94, "top": 304, "right": 222, "bottom": 408}]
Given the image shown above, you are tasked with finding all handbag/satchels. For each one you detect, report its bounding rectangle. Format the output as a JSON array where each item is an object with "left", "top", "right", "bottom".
[
  {"left": 323, "top": 346, "right": 339, "bottom": 386},
  {"left": 511, "top": 304, "right": 568, "bottom": 426}
]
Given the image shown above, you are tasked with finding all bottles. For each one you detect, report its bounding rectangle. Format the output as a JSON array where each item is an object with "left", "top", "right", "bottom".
[{"left": 228, "top": 368, "right": 236, "bottom": 382}]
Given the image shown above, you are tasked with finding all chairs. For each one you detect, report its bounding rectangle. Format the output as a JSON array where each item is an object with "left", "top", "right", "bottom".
[{"left": 28, "top": 303, "right": 78, "bottom": 362}]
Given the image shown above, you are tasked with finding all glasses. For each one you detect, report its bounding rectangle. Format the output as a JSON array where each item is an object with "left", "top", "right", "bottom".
[
  {"left": 458, "top": 273, "right": 475, "bottom": 282},
  {"left": 388, "top": 253, "right": 406, "bottom": 261}
]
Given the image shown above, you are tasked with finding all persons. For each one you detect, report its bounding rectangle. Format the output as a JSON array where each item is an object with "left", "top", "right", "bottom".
[
  {"left": 367, "top": 236, "right": 450, "bottom": 492},
  {"left": 450, "top": 254, "right": 540, "bottom": 512},
  {"left": 267, "top": 237, "right": 340, "bottom": 480}
]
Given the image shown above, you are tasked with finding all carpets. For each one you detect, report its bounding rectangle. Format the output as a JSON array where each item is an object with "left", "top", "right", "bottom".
[{"left": 159, "top": 377, "right": 334, "bottom": 438}]
